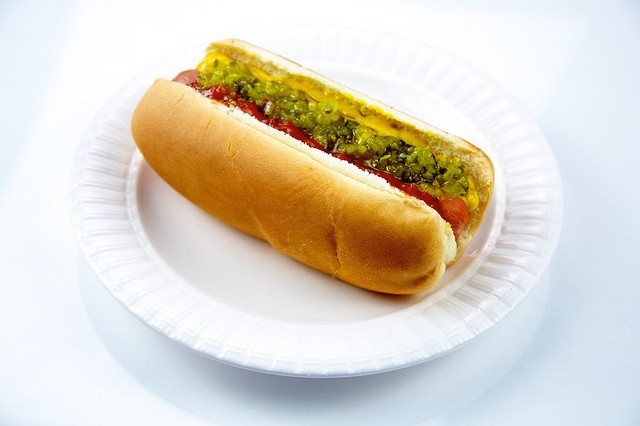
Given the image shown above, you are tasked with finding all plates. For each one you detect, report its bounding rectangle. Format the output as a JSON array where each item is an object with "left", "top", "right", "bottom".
[{"left": 68, "top": 39, "right": 565, "bottom": 377}]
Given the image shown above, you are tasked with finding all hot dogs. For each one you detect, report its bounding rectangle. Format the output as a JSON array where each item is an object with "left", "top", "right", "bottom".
[{"left": 128, "top": 36, "right": 495, "bottom": 297}]
